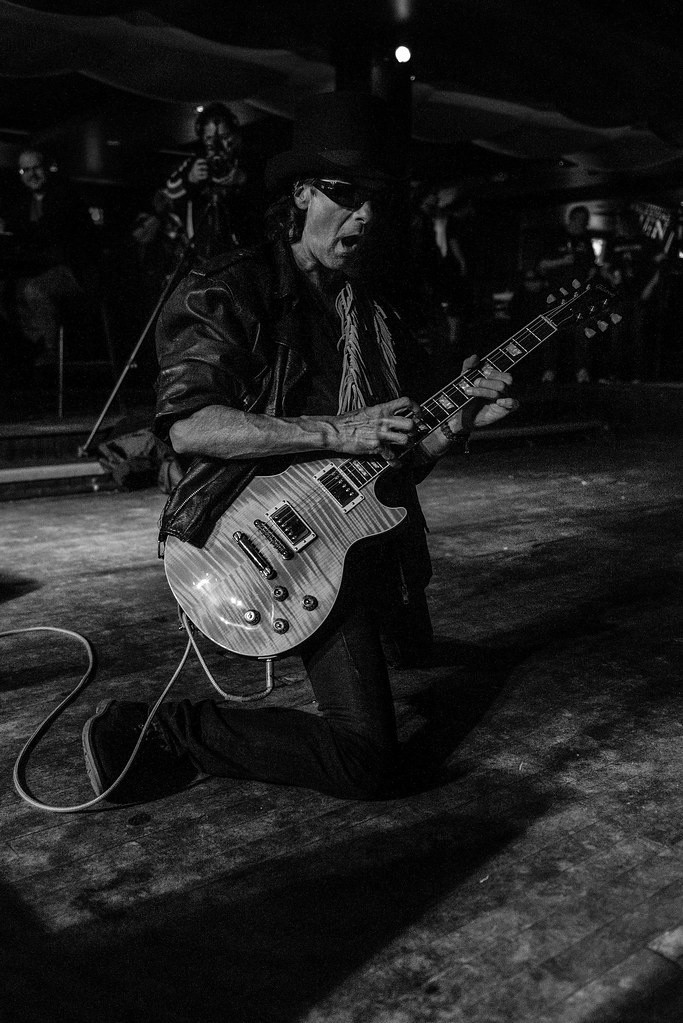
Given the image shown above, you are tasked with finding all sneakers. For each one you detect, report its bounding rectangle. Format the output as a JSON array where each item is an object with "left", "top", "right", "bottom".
[{"left": 82, "top": 699, "right": 162, "bottom": 798}]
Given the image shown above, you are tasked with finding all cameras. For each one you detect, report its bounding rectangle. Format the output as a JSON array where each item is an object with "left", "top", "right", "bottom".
[{"left": 201, "top": 153, "right": 233, "bottom": 183}]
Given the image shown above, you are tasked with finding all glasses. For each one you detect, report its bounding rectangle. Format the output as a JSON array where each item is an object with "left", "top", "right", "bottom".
[{"left": 302, "top": 176, "right": 392, "bottom": 219}]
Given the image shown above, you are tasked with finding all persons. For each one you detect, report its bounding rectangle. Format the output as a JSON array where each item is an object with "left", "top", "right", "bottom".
[
  {"left": 154, "top": 102, "right": 270, "bottom": 266},
  {"left": 81, "top": 140, "right": 519, "bottom": 802},
  {"left": 403, "top": 180, "right": 670, "bottom": 385},
  {"left": 12, "top": 149, "right": 107, "bottom": 369}
]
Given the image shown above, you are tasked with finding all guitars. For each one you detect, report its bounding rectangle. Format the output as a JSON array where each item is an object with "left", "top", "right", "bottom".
[{"left": 162, "top": 279, "right": 625, "bottom": 663}]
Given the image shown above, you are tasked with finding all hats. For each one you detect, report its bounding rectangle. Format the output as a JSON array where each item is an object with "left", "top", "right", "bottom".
[{"left": 266, "top": 92, "right": 452, "bottom": 208}]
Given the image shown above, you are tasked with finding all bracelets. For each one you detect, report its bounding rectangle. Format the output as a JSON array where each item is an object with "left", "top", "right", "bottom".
[{"left": 440, "top": 420, "right": 459, "bottom": 444}]
